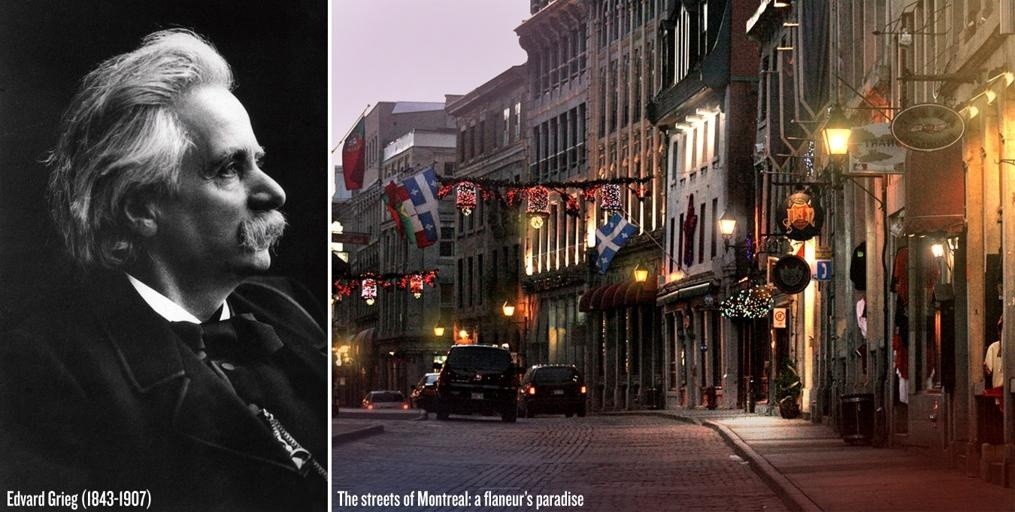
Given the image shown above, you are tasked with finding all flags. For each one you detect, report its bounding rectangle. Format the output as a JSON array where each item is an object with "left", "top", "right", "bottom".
[
  {"left": 341, "top": 115, "right": 366, "bottom": 191},
  {"left": 594, "top": 211, "right": 638, "bottom": 276},
  {"left": 380, "top": 165, "right": 442, "bottom": 250}
]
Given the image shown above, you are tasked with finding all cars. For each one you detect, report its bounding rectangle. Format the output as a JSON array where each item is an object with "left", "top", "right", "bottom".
[
  {"left": 359, "top": 388, "right": 409, "bottom": 413},
  {"left": 510, "top": 362, "right": 590, "bottom": 420},
  {"left": 409, "top": 370, "right": 443, "bottom": 414}
]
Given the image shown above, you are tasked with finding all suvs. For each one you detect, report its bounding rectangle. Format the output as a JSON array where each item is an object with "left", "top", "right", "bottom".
[{"left": 434, "top": 343, "right": 528, "bottom": 423}]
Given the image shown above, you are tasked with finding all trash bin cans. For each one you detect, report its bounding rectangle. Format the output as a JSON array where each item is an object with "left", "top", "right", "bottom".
[
  {"left": 646, "top": 388, "right": 660, "bottom": 409},
  {"left": 841, "top": 393, "right": 875, "bottom": 445}
]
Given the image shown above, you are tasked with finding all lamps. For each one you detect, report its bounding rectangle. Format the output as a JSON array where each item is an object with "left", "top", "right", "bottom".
[
  {"left": 717, "top": 199, "right": 754, "bottom": 235},
  {"left": 819, "top": 70, "right": 900, "bottom": 157},
  {"left": 634, "top": 255, "right": 656, "bottom": 282},
  {"left": 502, "top": 296, "right": 526, "bottom": 317}
]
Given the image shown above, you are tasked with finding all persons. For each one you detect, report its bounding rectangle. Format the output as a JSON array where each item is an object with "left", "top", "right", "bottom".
[{"left": 0, "top": 20, "right": 328, "bottom": 512}]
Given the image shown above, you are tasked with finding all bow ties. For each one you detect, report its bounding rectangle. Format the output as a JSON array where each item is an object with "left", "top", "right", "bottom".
[{"left": 165, "top": 309, "right": 290, "bottom": 374}]
